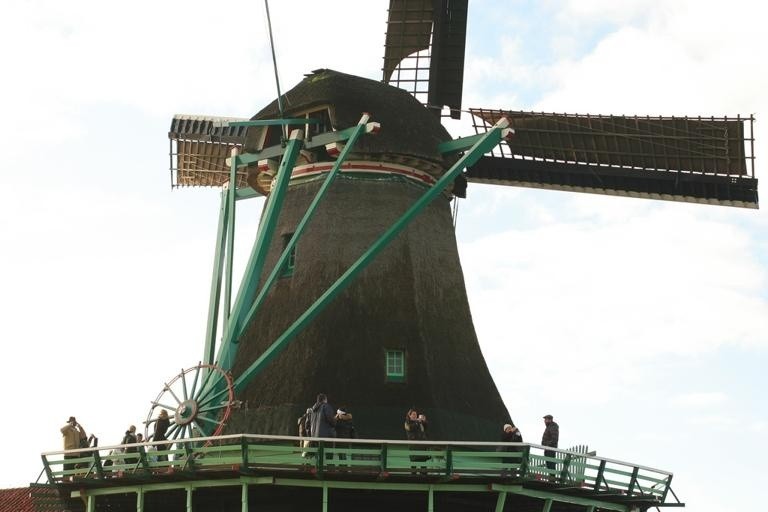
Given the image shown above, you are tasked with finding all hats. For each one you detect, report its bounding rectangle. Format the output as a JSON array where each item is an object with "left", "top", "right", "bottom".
[
  {"left": 336, "top": 407, "right": 347, "bottom": 415},
  {"left": 543, "top": 415, "right": 553, "bottom": 419},
  {"left": 504, "top": 423, "right": 512, "bottom": 432}
]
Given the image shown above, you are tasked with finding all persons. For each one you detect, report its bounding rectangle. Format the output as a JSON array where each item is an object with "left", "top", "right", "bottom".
[
  {"left": 332, "top": 405, "right": 352, "bottom": 467},
  {"left": 541, "top": 414, "right": 559, "bottom": 482},
  {"left": 134, "top": 431, "right": 150, "bottom": 469},
  {"left": 120, "top": 429, "right": 129, "bottom": 452},
  {"left": 151, "top": 409, "right": 170, "bottom": 467},
  {"left": 59, "top": 416, "right": 87, "bottom": 480},
  {"left": 405, "top": 408, "right": 433, "bottom": 474},
  {"left": 308, "top": 391, "right": 339, "bottom": 469},
  {"left": 123, "top": 424, "right": 139, "bottom": 475},
  {"left": 300, "top": 407, "right": 314, "bottom": 458},
  {"left": 500, "top": 423, "right": 523, "bottom": 479},
  {"left": 109, "top": 445, "right": 126, "bottom": 480},
  {"left": 297, "top": 412, "right": 307, "bottom": 451}
]
